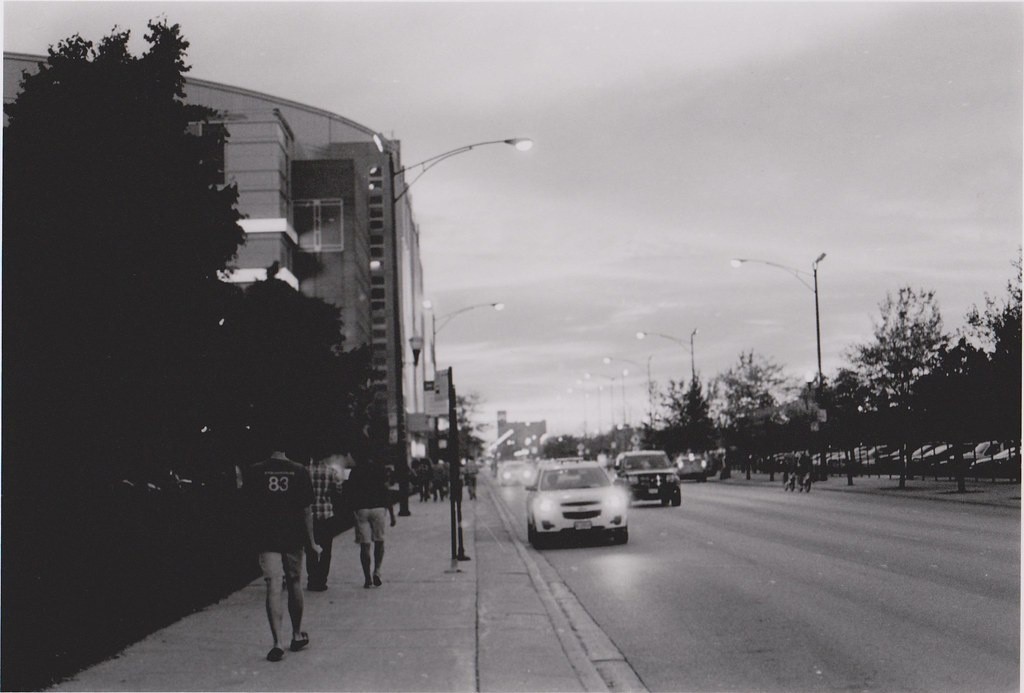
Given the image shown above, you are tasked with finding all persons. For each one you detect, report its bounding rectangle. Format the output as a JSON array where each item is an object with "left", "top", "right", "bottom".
[
  {"left": 779, "top": 449, "right": 811, "bottom": 490},
  {"left": 300, "top": 452, "right": 480, "bottom": 593},
  {"left": 249, "top": 440, "right": 323, "bottom": 658}
]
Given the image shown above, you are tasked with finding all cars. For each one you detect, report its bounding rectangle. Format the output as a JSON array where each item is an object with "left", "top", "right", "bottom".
[
  {"left": 674, "top": 457, "right": 708, "bottom": 483},
  {"left": 754, "top": 439, "right": 1021, "bottom": 480},
  {"left": 523, "top": 460, "right": 630, "bottom": 550},
  {"left": 613, "top": 450, "right": 682, "bottom": 508},
  {"left": 498, "top": 460, "right": 525, "bottom": 487}
]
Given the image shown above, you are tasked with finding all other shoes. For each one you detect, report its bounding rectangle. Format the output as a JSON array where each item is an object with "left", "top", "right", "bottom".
[
  {"left": 364, "top": 575, "right": 372, "bottom": 588},
  {"left": 290, "top": 632, "right": 309, "bottom": 652},
  {"left": 373, "top": 575, "right": 382, "bottom": 586},
  {"left": 307, "top": 583, "right": 327, "bottom": 591},
  {"left": 267, "top": 648, "right": 284, "bottom": 662}
]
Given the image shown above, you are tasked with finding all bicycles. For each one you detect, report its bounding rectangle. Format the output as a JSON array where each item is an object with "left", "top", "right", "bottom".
[
  {"left": 783, "top": 469, "right": 796, "bottom": 492},
  {"left": 798, "top": 467, "right": 812, "bottom": 493}
]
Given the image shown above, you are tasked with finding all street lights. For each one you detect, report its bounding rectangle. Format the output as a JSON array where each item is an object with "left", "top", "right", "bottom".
[
  {"left": 431, "top": 301, "right": 505, "bottom": 371},
  {"left": 386, "top": 135, "right": 534, "bottom": 519},
  {"left": 635, "top": 327, "right": 700, "bottom": 452},
  {"left": 728, "top": 252, "right": 829, "bottom": 481}
]
